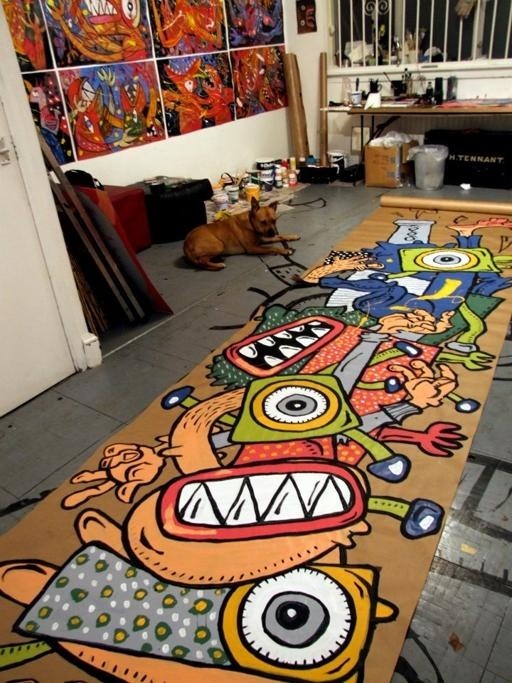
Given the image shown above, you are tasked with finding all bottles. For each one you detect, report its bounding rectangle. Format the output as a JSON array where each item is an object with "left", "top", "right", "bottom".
[
  {"left": 400, "top": 68, "right": 433, "bottom": 98},
  {"left": 275, "top": 154, "right": 321, "bottom": 189}
]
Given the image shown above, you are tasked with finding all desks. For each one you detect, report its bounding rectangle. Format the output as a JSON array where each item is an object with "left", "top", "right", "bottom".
[
  {"left": 347, "top": 98, "right": 512, "bottom": 187},
  {"left": 128, "top": 175, "right": 214, "bottom": 244}
]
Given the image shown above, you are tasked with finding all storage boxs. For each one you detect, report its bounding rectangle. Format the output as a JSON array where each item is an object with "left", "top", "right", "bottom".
[{"left": 364, "top": 140, "right": 419, "bottom": 188}]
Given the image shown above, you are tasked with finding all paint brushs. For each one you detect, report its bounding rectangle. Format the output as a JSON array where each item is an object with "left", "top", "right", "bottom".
[{"left": 401, "top": 67, "right": 411, "bottom": 84}]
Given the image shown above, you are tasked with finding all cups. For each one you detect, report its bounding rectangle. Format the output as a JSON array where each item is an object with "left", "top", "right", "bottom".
[
  {"left": 351, "top": 91, "right": 362, "bottom": 107},
  {"left": 369, "top": 92, "right": 381, "bottom": 108}
]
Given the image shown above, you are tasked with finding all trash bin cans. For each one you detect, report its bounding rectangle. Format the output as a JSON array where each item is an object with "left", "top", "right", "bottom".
[{"left": 408, "top": 144, "right": 449, "bottom": 190}]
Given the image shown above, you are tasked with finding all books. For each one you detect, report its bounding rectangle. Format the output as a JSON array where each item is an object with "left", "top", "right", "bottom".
[{"left": 383, "top": 98, "right": 419, "bottom": 107}]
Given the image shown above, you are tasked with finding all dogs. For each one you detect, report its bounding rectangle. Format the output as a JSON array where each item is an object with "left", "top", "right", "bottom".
[{"left": 183, "top": 195, "right": 301, "bottom": 271}]
